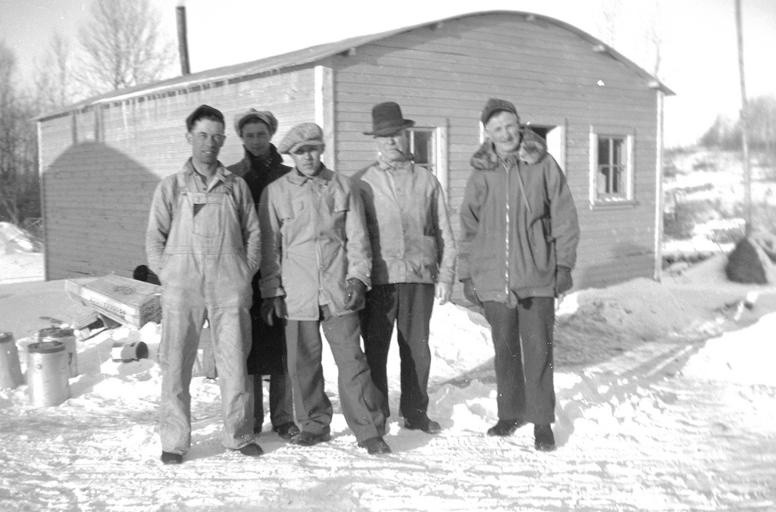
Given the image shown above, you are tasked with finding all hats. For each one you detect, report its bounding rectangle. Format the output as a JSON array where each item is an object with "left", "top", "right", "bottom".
[
  {"left": 233, "top": 107, "right": 277, "bottom": 137},
  {"left": 481, "top": 99, "right": 516, "bottom": 123},
  {"left": 279, "top": 122, "right": 324, "bottom": 154},
  {"left": 362, "top": 102, "right": 415, "bottom": 136},
  {"left": 186, "top": 105, "right": 224, "bottom": 131}
]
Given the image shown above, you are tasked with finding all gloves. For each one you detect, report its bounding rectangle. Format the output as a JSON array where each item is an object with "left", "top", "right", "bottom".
[
  {"left": 463, "top": 279, "right": 482, "bottom": 306},
  {"left": 259, "top": 297, "right": 284, "bottom": 327},
  {"left": 555, "top": 265, "right": 571, "bottom": 298},
  {"left": 344, "top": 278, "right": 366, "bottom": 312}
]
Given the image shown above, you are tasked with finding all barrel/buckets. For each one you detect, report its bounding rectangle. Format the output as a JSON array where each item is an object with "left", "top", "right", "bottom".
[
  {"left": 24, "top": 340, "right": 71, "bottom": 408},
  {"left": 0, "top": 331, "right": 24, "bottom": 391},
  {"left": 37, "top": 326, "right": 80, "bottom": 378}
]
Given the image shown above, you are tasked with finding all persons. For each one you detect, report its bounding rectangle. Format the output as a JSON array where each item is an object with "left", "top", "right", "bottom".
[
  {"left": 455, "top": 96, "right": 581, "bottom": 451},
  {"left": 143, "top": 103, "right": 263, "bottom": 461},
  {"left": 347, "top": 102, "right": 459, "bottom": 433},
  {"left": 222, "top": 107, "right": 296, "bottom": 438},
  {"left": 258, "top": 120, "right": 391, "bottom": 459}
]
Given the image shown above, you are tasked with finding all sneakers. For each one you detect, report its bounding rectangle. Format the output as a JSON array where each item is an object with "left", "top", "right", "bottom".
[
  {"left": 358, "top": 436, "right": 391, "bottom": 455},
  {"left": 162, "top": 451, "right": 182, "bottom": 465},
  {"left": 405, "top": 414, "right": 440, "bottom": 434},
  {"left": 254, "top": 426, "right": 262, "bottom": 433},
  {"left": 533, "top": 424, "right": 554, "bottom": 451},
  {"left": 488, "top": 419, "right": 526, "bottom": 436},
  {"left": 273, "top": 421, "right": 299, "bottom": 438},
  {"left": 241, "top": 443, "right": 263, "bottom": 457},
  {"left": 291, "top": 431, "right": 330, "bottom": 446}
]
